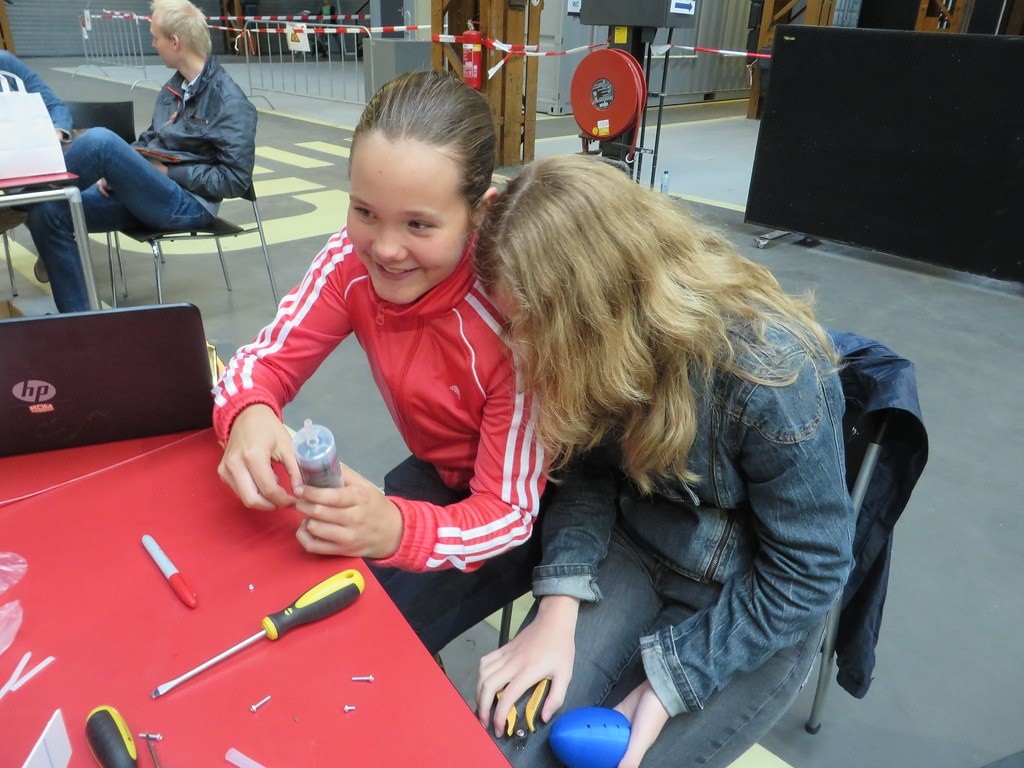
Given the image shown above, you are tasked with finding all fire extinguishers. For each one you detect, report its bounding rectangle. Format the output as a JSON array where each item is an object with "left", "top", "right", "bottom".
[{"left": 460, "top": 14, "right": 484, "bottom": 92}]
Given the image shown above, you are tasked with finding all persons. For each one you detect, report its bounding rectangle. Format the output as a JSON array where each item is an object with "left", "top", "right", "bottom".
[
  {"left": 27, "top": 0, "right": 259, "bottom": 313},
  {"left": 471, "top": 151, "right": 859, "bottom": 768},
  {"left": 210, "top": 68, "right": 568, "bottom": 655},
  {"left": 0, "top": 51, "right": 103, "bottom": 194}
]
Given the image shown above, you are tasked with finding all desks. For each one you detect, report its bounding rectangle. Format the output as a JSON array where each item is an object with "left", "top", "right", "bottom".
[
  {"left": 0, "top": 173, "right": 101, "bottom": 314},
  {"left": 0, "top": 366, "right": 515, "bottom": 767}
]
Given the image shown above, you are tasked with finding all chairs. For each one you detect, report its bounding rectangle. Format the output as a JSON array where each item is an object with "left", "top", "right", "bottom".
[
  {"left": 793, "top": 329, "right": 902, "bottom": 735},
  {"left": 64, "top": 100, "right": 137, "bottom": 310},
  {"left": 114, "top": 176, "right": 279, "bottom": 315}
]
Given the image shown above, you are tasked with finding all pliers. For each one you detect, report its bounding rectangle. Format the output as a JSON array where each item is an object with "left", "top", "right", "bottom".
[{"left": 494, "top": 679, "right": 552, "bottom": 750}]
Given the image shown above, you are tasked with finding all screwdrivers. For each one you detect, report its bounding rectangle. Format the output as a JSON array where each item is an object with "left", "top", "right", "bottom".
[
  {"left": 150, "top": 567, "right": 365, "bottom": 699},
  {"left": 84, "top": 705, "right": 140, "bottom": 768}
]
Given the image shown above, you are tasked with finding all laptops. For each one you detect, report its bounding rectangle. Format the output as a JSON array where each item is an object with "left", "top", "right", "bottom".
[{"left": 0, "top": 303, "right": 218, "bottom": 454}]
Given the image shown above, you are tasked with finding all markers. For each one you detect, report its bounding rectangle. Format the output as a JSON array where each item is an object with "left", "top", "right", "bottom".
[{"left": 141, "top": 534, "right": 198, "bottom": 609}]
